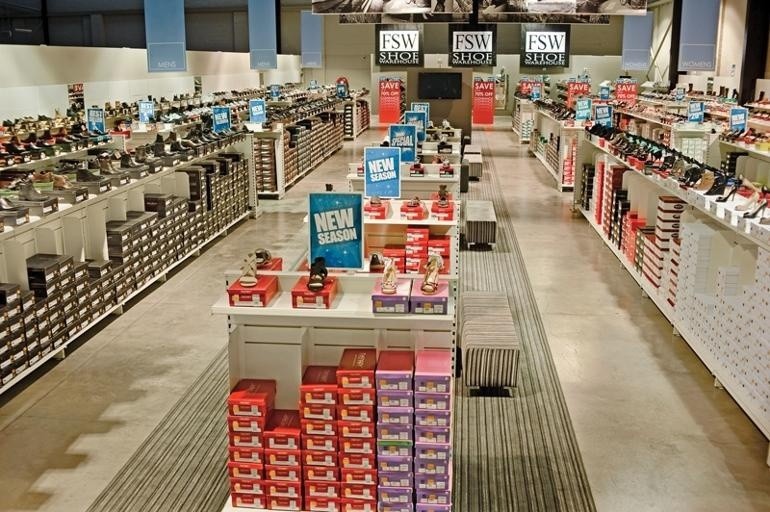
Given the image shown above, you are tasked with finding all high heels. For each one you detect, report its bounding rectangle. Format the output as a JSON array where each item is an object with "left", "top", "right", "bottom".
[{"left": 586, "top": 123, "right": 770, "bottom": 225}]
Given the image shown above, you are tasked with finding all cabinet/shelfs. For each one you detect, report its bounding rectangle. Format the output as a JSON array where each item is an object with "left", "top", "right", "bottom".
[
  {"left": 209, "top": 123, "right": 461, "bottom": 507},
  {"left": 512, "top": 77, "right": 770, "bottom": 191},
  {"left": 0, "top": 113, "right": 259, "bottom": 393},
  {"left": 571, "top": 125, "right": 770, "bottom": 438},
  {"left": 70, "top": 82, "right": 371, "bottom": 200}
]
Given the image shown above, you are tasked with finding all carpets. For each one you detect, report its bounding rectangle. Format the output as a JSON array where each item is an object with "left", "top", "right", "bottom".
[{"left": 85, "top": 142, "right": 598, "bottom": 512}]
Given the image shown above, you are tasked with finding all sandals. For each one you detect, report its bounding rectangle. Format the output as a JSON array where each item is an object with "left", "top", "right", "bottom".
[
  {"left": 239, "top": 252, "right": 257, "bottom": 287},
  {"left": 255, "top": 247, "right": 273, "bottom": 268},
  {"left": 369, "top": 250, "right": 386, "bottom": 269},
  {"left": 421, "top": 257, "right": 441, "bottom": 294},
  {"left": 370, "top": 195, "right": 382, "bottom": 207},
  {"left": 307, "top": 257, "right": 328, "bottom": 292},
  {"left": 380, "top": 258, "right": 398, "bottom": 294},
  {"left": 407, "top": 195, "right": 419, "bottom": 206},
  {"left": 437, "top": 184, "right": 450, "bottom": 208}
]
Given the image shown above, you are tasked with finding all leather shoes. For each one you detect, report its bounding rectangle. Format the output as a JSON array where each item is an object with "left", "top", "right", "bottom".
[
  {"left": 1, "top": 92, "right": 245, "bottom": 210},
  {"left": 514, "top": 80, "right": 576, "bottom": 121},
  {"left": 242, "top": 78, "right": 366, "bottom": 136}
]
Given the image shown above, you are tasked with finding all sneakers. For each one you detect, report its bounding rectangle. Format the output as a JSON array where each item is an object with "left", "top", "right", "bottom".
[{"left": 413, "top": 120, "right": 451, "bottom": 168}]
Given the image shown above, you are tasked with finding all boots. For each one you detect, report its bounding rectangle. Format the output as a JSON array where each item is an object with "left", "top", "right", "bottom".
[{"left": 612, "top": 84, "right": 770, "bottom": 124}]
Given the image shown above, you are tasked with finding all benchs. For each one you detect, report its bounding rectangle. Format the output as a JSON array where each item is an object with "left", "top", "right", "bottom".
[
  {"left": 461, "top": 293, "right": 520, "bottom": 396},
  {"left": 463, "top": 144, "right": 486, "bottom": 178},
  {"left": 466, "top": 199, "right": 497, "bottom": 250}
]
